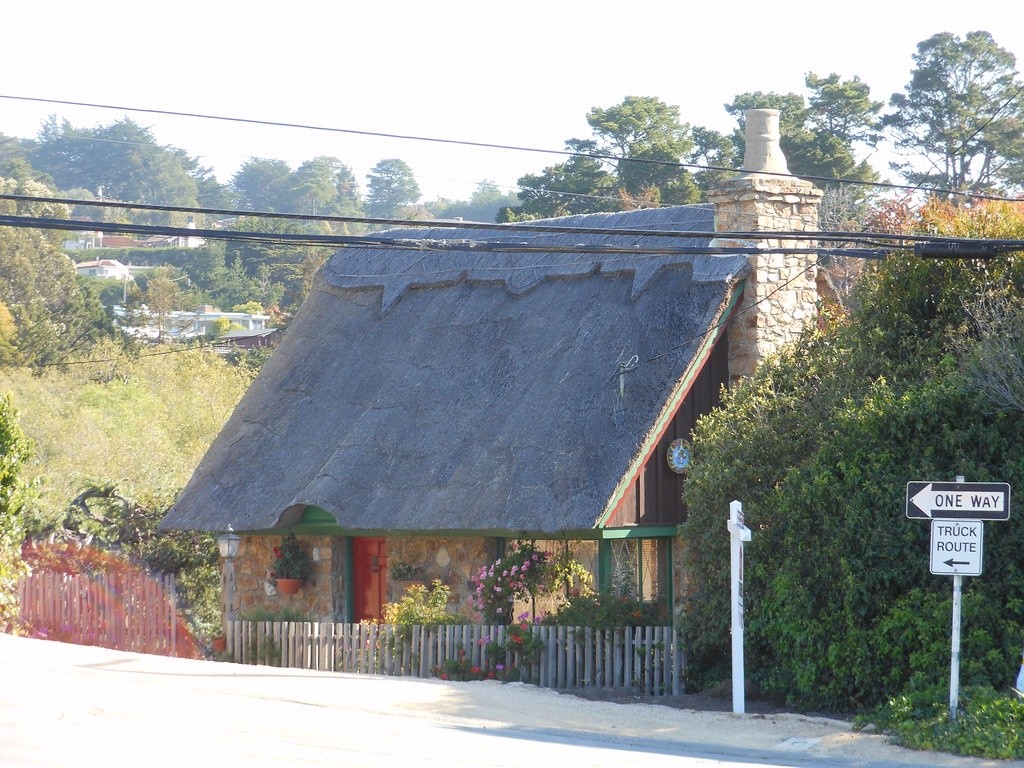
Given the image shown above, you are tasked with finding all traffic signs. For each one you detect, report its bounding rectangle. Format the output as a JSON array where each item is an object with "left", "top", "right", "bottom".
[{"left": 905, "top": 478, "right": 1013, "bottom": 521}]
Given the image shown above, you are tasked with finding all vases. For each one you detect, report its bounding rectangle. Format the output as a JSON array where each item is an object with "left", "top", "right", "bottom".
[
  {"left": 275, "top": 577, "right": 303, "bottom": 595},
  {"left": 400, "top": 580, "right": 424, "bottom": 591},
  {"left": 212, "top": 636, "right": 226, "bottom": 653}
]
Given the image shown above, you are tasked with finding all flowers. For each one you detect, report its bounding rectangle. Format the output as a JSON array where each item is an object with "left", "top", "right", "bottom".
[
  {"left": 385, "top": 562, "right": 426, "bottom": 582},
  {"left": 267, "top": 533, "right": 311, "bottom": 592}
]
